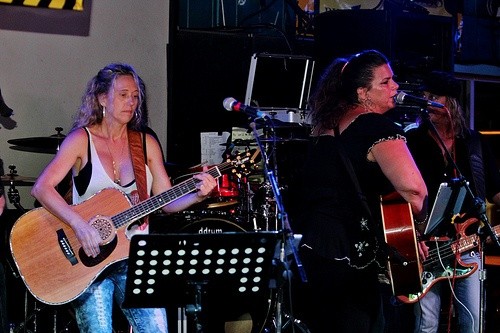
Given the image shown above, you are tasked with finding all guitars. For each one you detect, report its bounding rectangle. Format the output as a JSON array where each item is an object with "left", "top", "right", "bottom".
[
  {"left": 7, "top": 147, "right": 263, "bottom": 306},
  {"left": 398, "top": 216, "right": 500, "bottom": 305},
  {"left": 378, "top": 179, "right": 425, "bottom": 297}
]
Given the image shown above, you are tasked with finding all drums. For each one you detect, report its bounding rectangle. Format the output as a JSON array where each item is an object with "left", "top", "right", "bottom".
[{"left": 177, "top": 165, "right": 278, "bottom": 233}]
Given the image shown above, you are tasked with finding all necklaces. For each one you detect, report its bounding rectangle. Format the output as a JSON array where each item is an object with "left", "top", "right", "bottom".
[{"left": 100, "top": 127, "right": 126, "bottom": 183}]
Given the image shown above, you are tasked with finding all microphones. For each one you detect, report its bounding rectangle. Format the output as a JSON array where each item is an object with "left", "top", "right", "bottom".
[
  {"left": 396, "top": 91, "right": 445, "bottom": 109},
  {"left": 223, "top": 97, "right": 265, "bottom": 117}
]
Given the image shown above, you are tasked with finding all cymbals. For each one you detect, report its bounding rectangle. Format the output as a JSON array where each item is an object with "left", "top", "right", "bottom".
[
  {"left": 0, "top": 174, "right": 38, "bottom": 180},
  {"left": 8, "top": 133, "right": 66, "bottom": 146},
  {"left": 257, "top": 118, "right": 310, "bottom": 129},
  {"left": 220, "top": 135, "right": 311, "bottom": 146}
]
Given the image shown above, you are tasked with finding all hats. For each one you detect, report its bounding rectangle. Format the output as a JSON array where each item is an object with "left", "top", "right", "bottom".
[{"left": 414, "top": 70, "right": 462, "bottom": 99}]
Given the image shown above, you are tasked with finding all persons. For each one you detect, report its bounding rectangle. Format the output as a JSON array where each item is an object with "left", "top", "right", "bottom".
[
  {"left": 32, "top": 63, "right": 219, "bottom": 333},
  {"left": 403, "top": 72, "right": 500, "bottom": 333},
  {"left": 304, "top": 48, "right": 429, "bottom": 333}
]
